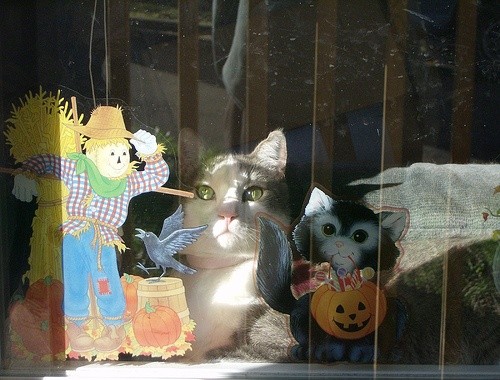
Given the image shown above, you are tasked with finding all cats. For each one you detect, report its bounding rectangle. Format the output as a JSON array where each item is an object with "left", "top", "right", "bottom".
[{"left": 130, "top": 128, "right": 298, "bottom": 363}]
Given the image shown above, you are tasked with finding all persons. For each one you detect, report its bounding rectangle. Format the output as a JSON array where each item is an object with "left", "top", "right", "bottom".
[{"left": 210, "top": 0, "right": 424, "bottom": 180}]
